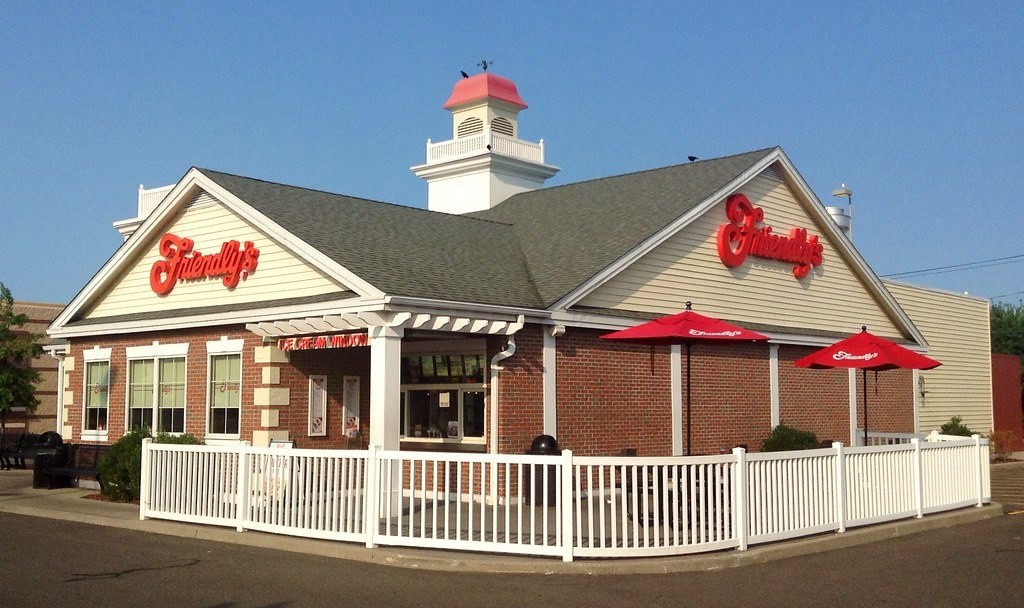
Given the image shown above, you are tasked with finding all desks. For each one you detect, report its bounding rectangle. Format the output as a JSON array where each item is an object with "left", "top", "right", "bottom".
[{"left": 642, "top": 466, "right": 718, "bottom": 531}]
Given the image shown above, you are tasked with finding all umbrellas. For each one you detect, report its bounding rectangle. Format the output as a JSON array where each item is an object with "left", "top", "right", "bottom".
[
  {"left": 597, "top": 301, "right": 772, "bottom": 454},
  {"left": 793, "top": 326, "right": 944, "bottom": 447}
]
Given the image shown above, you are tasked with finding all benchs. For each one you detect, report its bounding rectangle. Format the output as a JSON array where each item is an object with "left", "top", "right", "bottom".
[{"left": 47, "top": 443, "right": 111, "bottom": 490}]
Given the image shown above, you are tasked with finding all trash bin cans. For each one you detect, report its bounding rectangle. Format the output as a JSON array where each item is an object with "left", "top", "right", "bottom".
[
  {"left": 32, "top": 431, "right": 63, "bottom": 489},
  {"left": 526, "top": 434, "right": 559, "bottom": 508}
]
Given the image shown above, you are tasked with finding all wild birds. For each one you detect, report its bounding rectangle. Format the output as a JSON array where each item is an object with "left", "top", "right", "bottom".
[
  {"left": 687, "top": 155, "right": 699, "bottom": 163},
  {"left": 460, "top": 71, "right": 470, "bottom": 79},
  {"left": 486, "top": 145, "right": 492, "bottom": 151}
]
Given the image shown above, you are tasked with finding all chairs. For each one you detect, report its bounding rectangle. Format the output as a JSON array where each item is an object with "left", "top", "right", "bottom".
[
  {"left": 617, "top": 448, "right": 667, "bottom": 527},
  {"left": 0, "top": 432, "right": 40, "bottom": 470}
]
